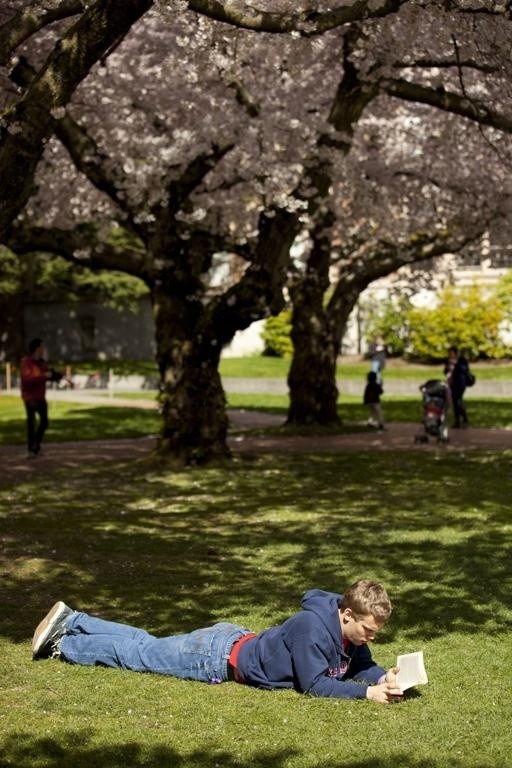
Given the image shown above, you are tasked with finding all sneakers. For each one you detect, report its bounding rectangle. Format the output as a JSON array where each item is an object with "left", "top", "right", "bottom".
[{"left": 31, "top": 601, "right": 74, "bottom": 655}]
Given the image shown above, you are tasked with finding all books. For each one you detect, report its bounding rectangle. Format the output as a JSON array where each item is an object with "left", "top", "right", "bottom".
[{"left": 393, "top": 651, "right": 429, "bottom": 693}]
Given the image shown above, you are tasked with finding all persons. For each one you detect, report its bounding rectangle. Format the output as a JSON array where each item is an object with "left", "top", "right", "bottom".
[
  {"left": 20, "top": 338, "right": 59, "bottom": 459},
  {"left": 363, "top": 371, "right": 386, "bottom": 429},
  {"left": 370, "top": 336, "right": 386, "bottom": 385},
  {"left": 31, "top": 580, "right": 402, "bottom": 705},
  {"left": 443, "top": 346, "right": 470, "bottom": 430}
]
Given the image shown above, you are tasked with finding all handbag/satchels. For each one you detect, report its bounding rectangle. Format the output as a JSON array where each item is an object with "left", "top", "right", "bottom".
[{"left": 466, "top": 372, "right": 475, "bottom": 386}]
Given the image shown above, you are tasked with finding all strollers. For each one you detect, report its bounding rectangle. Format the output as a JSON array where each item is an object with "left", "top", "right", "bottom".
[{"left": 415, "top": 381, "right": 449, "bottom": 445}]
